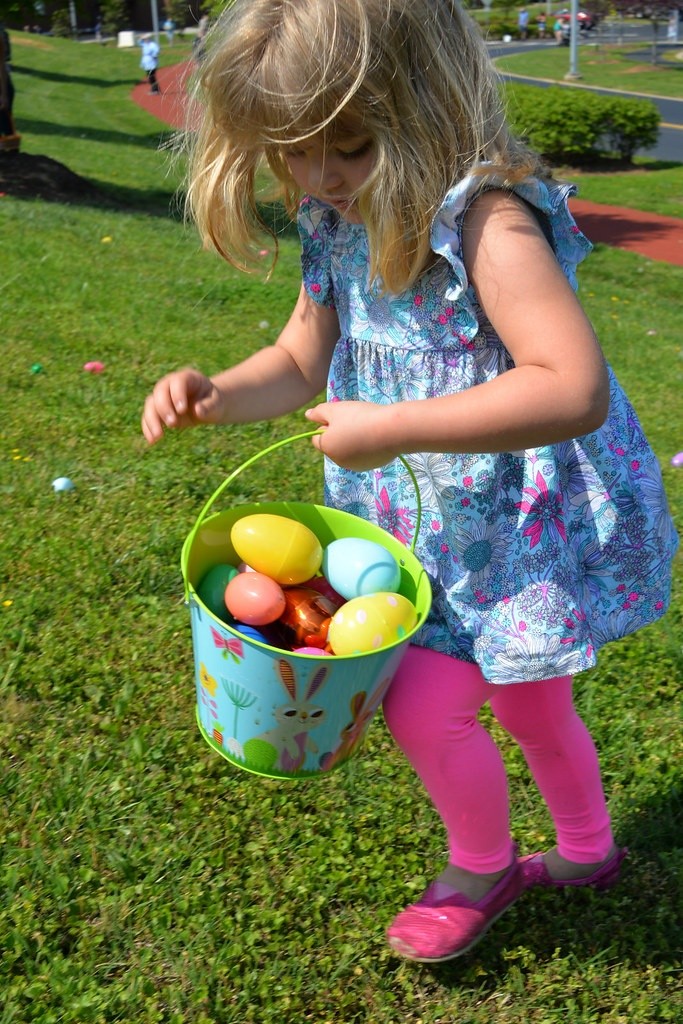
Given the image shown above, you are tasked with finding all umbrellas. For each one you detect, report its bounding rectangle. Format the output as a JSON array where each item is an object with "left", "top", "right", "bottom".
[{"left": 551, "top": 10, "right": 592, "bottom": 23}]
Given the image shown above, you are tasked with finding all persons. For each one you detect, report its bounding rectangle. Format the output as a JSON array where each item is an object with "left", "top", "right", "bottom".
[
  {"left": 141, "top": 0, "right": 679, "bottom": 962},
  {"left": 551, "top": 19, "right": 565, "bottom": 44},
  {"left": 161, "top": 15, "right": 176, "bottom": 48},
  {"left": 196, "top": 9, "right": 211, "bottom": 38},
  {"left": 517, "top": 9, "right": 528, "bottom": 43},
  {"left": 140, "top": 34, "right": 161, "bottom": 93},
  {"left": 667, "top": 17, "right": 678, "bottom": 41},
  {"left": 96, "top": 23, "right": 105, "bottom": 43},
  {"left": 536, "top": 11, "right": 547, "bottom": 38}
]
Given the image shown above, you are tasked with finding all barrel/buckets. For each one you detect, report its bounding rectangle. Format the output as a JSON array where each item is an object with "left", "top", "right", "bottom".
[{"left": 181, "top": 430, "right": 433, "bottom": 781}]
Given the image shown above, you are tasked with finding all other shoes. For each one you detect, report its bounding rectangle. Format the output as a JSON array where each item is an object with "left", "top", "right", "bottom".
[
  {"left": 519, "top": 834, "right": 629, "bottom": 885},
  {"left": 388, "top": 857, "right": 524, "bottom": 963}
]
